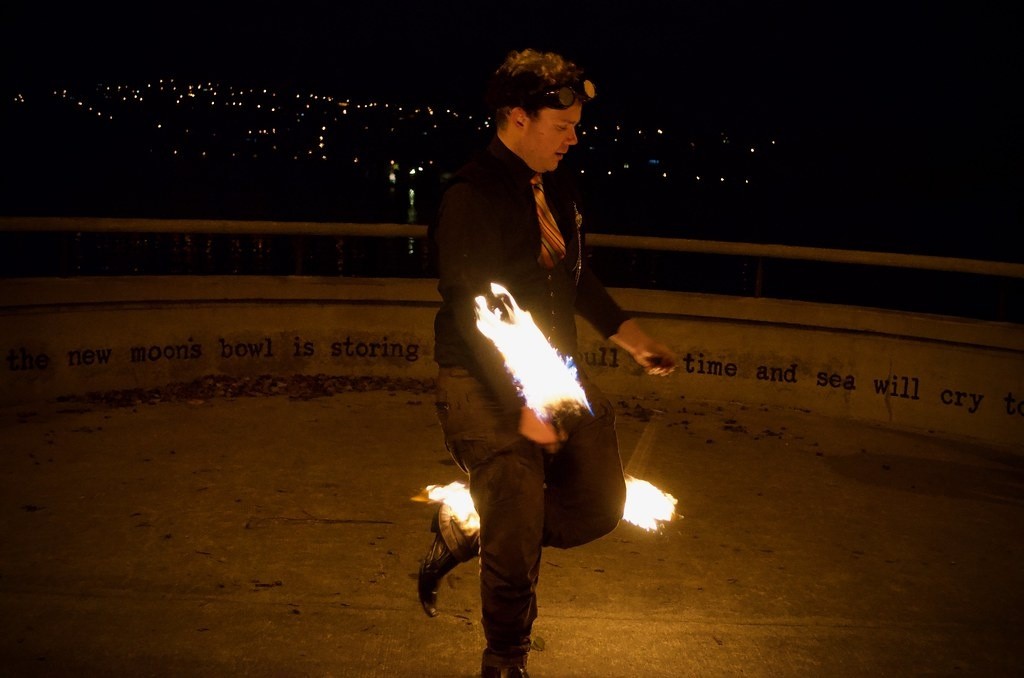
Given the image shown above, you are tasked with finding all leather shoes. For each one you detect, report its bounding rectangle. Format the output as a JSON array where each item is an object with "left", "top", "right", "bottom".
[
  {"left": 418, "top": 511, "right": 460, "bottom": 617},
  {"left": 481, "top": 666, "right": 529, "bottom": 678}
]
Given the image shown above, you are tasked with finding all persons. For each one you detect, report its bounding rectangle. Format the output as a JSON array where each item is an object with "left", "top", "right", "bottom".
[{"left": 416, "top": 43, "right": 682, "bottom": 678}]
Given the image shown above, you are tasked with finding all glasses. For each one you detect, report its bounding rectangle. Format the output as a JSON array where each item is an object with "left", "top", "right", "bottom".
[{"left": 529, "top": 79, "right": 596, "bottom": 110}]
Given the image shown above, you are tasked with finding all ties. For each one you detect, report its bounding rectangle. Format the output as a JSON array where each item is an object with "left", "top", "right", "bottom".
[{"left": 530, "top": 172, "right": 566, "bottom": 270}]
